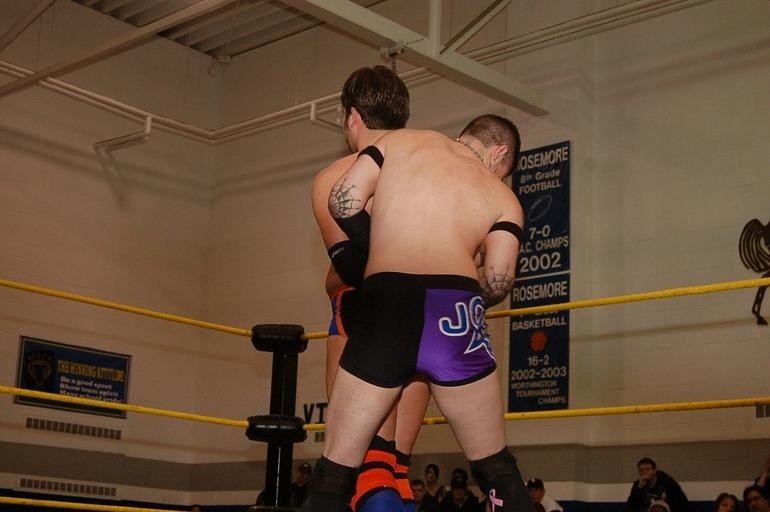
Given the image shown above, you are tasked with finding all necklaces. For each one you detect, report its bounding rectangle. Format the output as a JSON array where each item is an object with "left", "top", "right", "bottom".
[{"left": 450, "top": 136, "right": 485, "bottom": 166}]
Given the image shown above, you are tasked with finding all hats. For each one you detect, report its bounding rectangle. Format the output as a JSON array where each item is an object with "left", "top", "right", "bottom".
[
  {"left": 298, "top": 462, "right": 312, "bottom": 476},
  {"left": 649, "top": 501, "right": 670, "bottom": 511},
  {"left": 525, "top": 478, "right": 544, "bottom": 490}
]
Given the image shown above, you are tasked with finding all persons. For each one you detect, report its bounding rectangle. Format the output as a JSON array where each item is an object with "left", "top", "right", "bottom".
[
  {"left": 311, "top": 65, "right": 432, "bottom": 512},
  {"left": 308, "top": 113, "right": 537, "bottom": 512},
  {"left": 410, "top": 464, "right": 564, "bottom": 512},
  {"left": 623, "top": 457, "right": 770, "bottom": 512}
]
